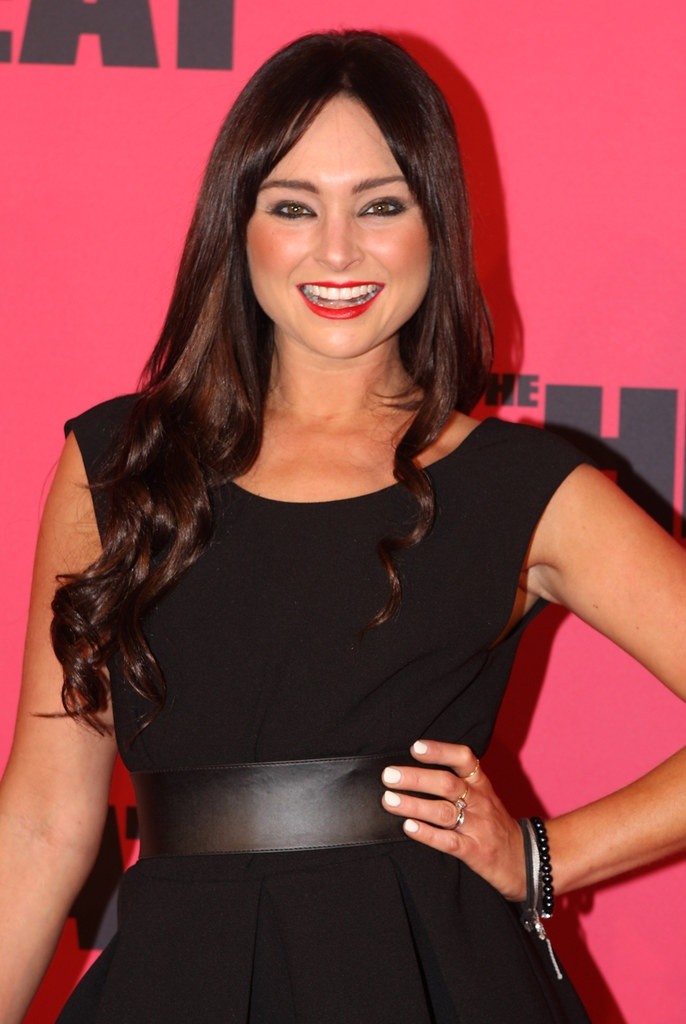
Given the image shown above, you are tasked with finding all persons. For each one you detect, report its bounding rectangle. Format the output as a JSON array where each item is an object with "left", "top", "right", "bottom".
[{"left": 1, "top": 29, "right": 686, "bottom": 1023}]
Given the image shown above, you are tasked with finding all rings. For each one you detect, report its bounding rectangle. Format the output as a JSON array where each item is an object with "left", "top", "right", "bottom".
[
  {"left": 450, "top": 785, "right": 469, "bottom": 831},
  {"left": 463, "top": 759, "right": 480, "bottom": 779}
]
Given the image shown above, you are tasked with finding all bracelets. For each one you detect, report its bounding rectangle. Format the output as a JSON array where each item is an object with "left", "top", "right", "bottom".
[{"left": 518, "top": 817, "right": 563, "bottom": 981}]
